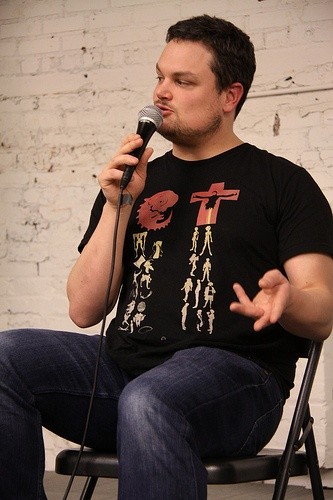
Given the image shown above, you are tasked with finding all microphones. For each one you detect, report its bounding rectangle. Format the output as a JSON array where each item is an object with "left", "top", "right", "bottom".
[{"left": 119, "top": 106, "right": 164, "bottom": 189}]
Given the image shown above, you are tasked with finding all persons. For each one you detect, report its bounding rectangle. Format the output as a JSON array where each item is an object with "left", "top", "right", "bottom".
[{"left": 1, "top": 13, "right": 333, "bottom": 500}]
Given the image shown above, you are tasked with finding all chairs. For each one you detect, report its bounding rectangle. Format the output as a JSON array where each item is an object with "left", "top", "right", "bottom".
[{"left": 55, "top": 336, "right": 327, "bottom": 500}]
